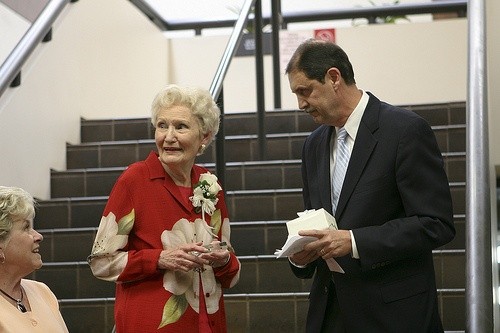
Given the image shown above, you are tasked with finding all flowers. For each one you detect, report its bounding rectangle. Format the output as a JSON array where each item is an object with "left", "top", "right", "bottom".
[{"left": 188, "top": 170, "right": 223, "bottom": 238}]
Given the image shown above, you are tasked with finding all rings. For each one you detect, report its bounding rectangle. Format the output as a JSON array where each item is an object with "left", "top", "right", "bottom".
[
  {"left": 209, "top": 261, "right": 214, "bottom": 266},
  {"left": 320, "top": 248, "right": 326, "bottom": 256}
]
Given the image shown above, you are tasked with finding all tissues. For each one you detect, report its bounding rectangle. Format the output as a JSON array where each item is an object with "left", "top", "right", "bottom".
[{"left": 285, "top": 208, "right": 338, "bottom": 235}]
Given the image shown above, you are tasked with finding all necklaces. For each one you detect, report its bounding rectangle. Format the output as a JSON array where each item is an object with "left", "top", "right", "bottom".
[{"left": 0, "top": 288, "right": 27, "bottom": 313}]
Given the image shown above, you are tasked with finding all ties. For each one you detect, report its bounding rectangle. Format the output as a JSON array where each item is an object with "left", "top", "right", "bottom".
[{"left": 331, "top": 125, "right": 350, "bottom": 218}]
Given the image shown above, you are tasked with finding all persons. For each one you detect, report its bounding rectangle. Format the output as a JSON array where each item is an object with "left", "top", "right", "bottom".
[
  {"left": 0, "top": 185, "right": 68, "bottom": 333},
  {"left": 283, "top": 38, "right": 455, "bottom": 333},
  {"left": 86, "top": 83, "right": 241, "bottom": 333}
]
